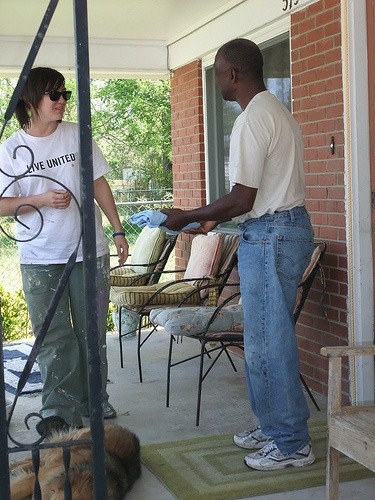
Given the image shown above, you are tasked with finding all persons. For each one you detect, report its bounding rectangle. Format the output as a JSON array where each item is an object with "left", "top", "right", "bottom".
[
  {"left": 0, "top": 65, "right": 129, "bottom": 439},
  {"left": 160, "top": 38, "right": 314, "bottom": 470}
]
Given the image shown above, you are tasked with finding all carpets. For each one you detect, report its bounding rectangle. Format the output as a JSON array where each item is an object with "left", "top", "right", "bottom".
[
  {"left": 139, "top": 420, "right": 375, "bottom": 500},
  {"left": 2, "top": 342, "right": 113, "bottom": 397}
]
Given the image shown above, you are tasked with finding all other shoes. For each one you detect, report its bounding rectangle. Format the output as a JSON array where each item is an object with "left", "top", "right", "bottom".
[
  {"left": 97, "top": 397, "right": 117, "bottom": 418},
  {"left": 36, "top": 409, "right": 84, "bottom": 437}
]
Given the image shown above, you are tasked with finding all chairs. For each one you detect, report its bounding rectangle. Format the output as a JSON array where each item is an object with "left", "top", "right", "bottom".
[
  {"left": 149, "top": 240, "right": 327, "bottom": 428},
  {"left": 109, "top": 231, "right": 241, "bottom": 384},
  {"left": 319, "top": 341, "right": 375, "bottom": 500},
  {"left": 108, "top": 225, "right": 179, "bottom": 342}
]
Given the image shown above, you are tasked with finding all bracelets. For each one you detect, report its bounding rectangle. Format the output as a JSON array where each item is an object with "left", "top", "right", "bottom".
[{"left": 113, "top": 232, "right": 125, "bottom": 237}]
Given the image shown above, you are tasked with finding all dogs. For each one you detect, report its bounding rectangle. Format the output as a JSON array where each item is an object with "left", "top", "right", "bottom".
[{"left": 8, "top": 423, "right": 143, "bottom": 500}]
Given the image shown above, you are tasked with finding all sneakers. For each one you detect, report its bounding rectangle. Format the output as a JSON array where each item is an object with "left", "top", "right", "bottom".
[
  {"left": 233, "top": 425, "right": 275, "bottom": 451},
  {"left": 243, "top": 436, "right": 316, "bottom": 471}
]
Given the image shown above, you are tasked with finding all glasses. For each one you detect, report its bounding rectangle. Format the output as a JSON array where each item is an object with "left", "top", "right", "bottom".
[{"left": 43, "top": 89, "right": 72, "bottom": 102}]
